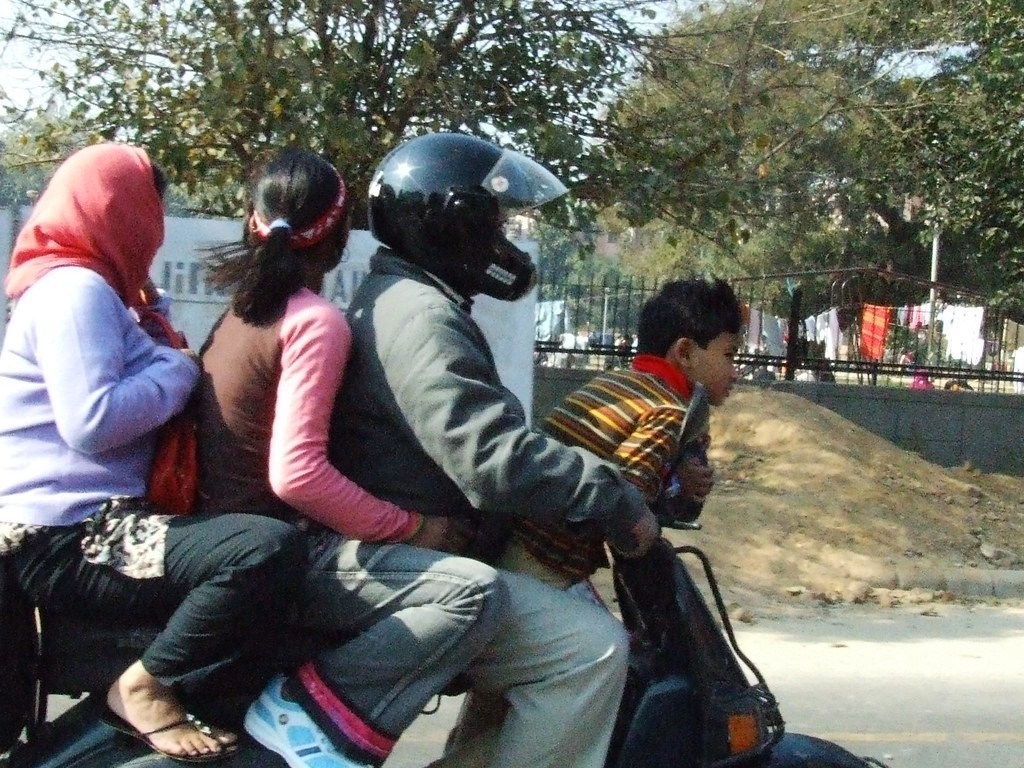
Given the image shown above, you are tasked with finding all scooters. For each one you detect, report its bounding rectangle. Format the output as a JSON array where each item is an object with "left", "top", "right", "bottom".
[{"left": 0, "top": 380, "right": 885, "bottom": 768}]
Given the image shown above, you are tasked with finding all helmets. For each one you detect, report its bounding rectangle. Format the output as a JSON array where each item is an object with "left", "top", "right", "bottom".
[{"left": 368, "top": 134, "right": 570, "bottom": 301}]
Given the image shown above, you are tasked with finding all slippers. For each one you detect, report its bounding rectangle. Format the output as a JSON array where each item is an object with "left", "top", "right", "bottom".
[{"left": 100, "top": 705, "right": 238, "bottom": 760}]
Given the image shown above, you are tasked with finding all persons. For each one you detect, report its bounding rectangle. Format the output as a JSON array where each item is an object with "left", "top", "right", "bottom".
[
  {"left": 557, "top": 281, "right": 593, "bottom": 342},
  {"left": 325, "top": 131, "right": 660, "bottom": 768},
  {"left": 193, "top": 146, "right": 510, "bottom": 768},
  {"left": 0, "top": 142, "right": 310, "bottom": 762},
  {"left": 503, "top": 276, "right": 746, "bottom": 768},
  {"left": 945, "top": 360, "right": 976, "bottom": 391}
]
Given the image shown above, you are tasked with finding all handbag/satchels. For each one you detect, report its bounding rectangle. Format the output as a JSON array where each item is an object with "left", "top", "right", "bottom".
[{"left": 139, "top": 313, "right": 196, "bottom": 515}]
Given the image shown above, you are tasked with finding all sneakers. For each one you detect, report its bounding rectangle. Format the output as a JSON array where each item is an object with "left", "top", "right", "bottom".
[{"left": 241, "top": 673, "right": 372, "bottom": 768}]
